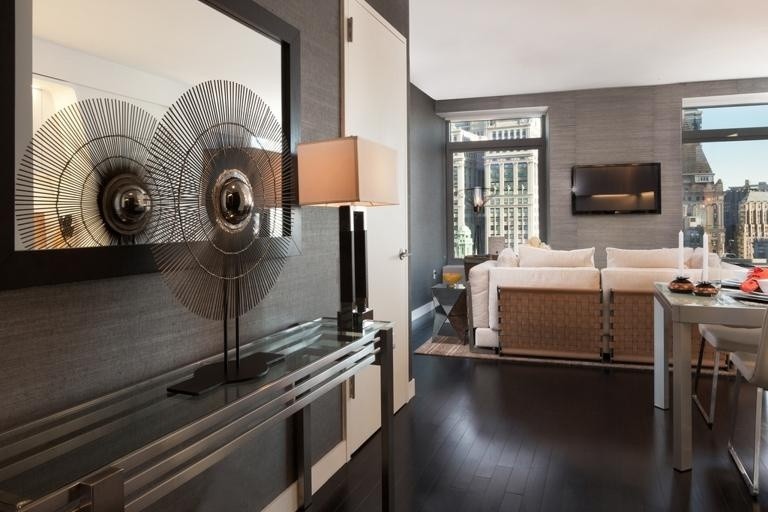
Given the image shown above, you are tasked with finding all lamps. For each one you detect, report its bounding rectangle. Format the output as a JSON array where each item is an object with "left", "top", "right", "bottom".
[
  {"left": 296, "top": 133, "right": 402, "bottom": 334},
  {"left": 198, "top": 144, "right": 295, "bottom": 241}
]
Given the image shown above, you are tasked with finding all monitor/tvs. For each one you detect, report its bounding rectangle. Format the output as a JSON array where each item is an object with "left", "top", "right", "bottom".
[{"left": 568, "top": 161, "right": 662, "bottom": 217}]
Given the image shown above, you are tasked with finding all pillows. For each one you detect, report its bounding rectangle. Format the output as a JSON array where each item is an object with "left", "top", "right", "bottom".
[
  {"left": 519, "top": 245, "right": 595, "bottom": 268},
  {"left": 689, "top": 244, "right": 722, "bottom": 269},
  {"left": 605, "top": 246, "right": 695, "bottom": 270},
  {"left": 496, "top": 247, "right": 518, "bottom": 266}
]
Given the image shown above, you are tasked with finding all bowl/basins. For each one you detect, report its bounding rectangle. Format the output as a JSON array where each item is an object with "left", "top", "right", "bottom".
[
  {"left": 442, "top": 274, "right": 462, "bottom": 283},
  {"left": 755, "top": 280, "right": 768, "bottom": 295}
]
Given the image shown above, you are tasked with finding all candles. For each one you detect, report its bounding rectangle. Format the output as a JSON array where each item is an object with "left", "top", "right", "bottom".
[
  {"left": 702, "top": 231, "right": 709, "bottom": 283},
  {"left": 678, "top": 229, "right": 685, "bottom": 278}
]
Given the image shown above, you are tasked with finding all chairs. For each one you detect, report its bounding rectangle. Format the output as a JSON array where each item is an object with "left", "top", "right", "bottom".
[{"left": 691, "top": 307, "right": 768, "bottom": 497}]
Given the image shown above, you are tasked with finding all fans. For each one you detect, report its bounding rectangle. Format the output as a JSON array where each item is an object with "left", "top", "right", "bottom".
[
  {"left": 138, "top": 79, "right": 304, "bottom": 398},
  {"left": 14, "top": 96, "right": 200, "bottom": 276}
]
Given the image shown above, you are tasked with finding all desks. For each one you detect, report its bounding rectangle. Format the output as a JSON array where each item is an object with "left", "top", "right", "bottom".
[
  {"left": 430, "top": 282, "right": 467, "bottom": 345},
  {"left": 652, "top": 280, "right": 768, "bottom": 472}
]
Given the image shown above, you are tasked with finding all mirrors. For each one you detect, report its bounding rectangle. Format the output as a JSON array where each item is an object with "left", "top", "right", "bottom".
[{"left": 3, "top": 1, "right": 302, "bottom": 289}]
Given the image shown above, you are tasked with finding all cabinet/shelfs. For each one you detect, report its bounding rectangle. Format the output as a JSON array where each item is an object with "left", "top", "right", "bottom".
[{"left": 0, "top": 320, "right": 400, "bottom": 512}]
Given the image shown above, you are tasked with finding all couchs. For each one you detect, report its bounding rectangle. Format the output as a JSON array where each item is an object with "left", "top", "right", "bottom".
[{"left": 467, "top": 258, "right": 754, "bottom": 378}]
